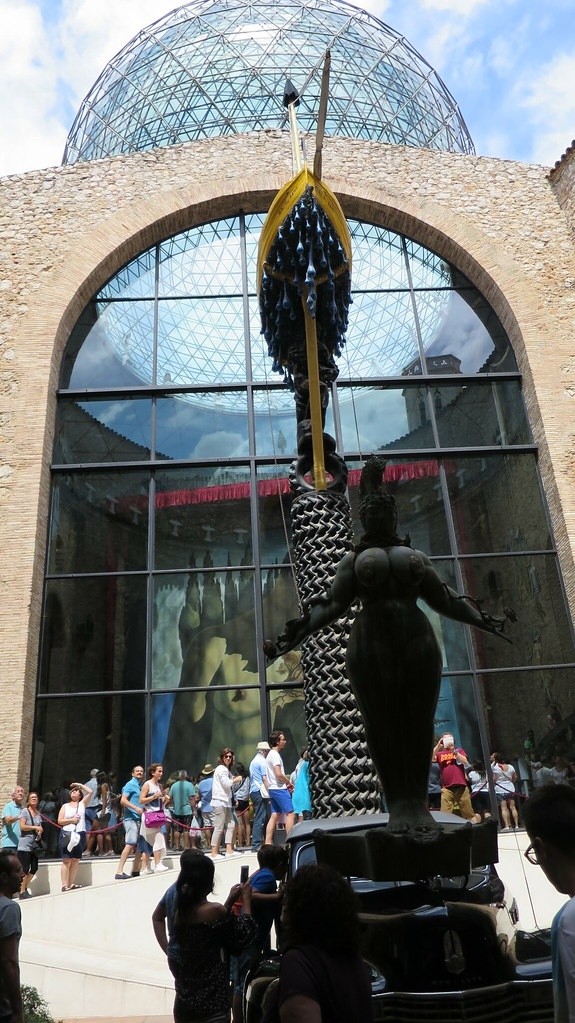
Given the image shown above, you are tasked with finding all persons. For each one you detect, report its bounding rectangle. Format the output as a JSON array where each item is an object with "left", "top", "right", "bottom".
[
  {"left": 428, "top": 713, "right": 575, "bottom": 833},
  {"left": 1, "top": 731, "right": 313, "bottom": 900},
  {"left": 0, "top": 848, "right": 25, "bottom": 1023},
  {"left": 152, "top": 843, "right": 372, "bottom": 1023},
  {"left": 265, "top": 456, "right": 515, "bottom": 833},
  {"left": 519, "top": 781, "right": 575, "bottom": 1023}
]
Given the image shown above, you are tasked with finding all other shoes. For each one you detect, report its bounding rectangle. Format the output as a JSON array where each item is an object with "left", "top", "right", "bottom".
[
  {"left": 82, "top": 852, "right": 91, "bottom": 856},
  {"left": 104, "top": 850, "right": 115, "bottom": 856},
  {"left": 115, "top": 873, "right": 132, "bottom": 879},
  {"left": 62, "top": 886, "right": 71, "bottom": 892},
  {"left": 19, "top": 891, "right": 32, "bottom": 900},
  {"left": 140, "top": 866, "right": 154, "bottom": 875},
  {"left": 500, "top": 828, "right": 510, "bottom": 833},
  {"left": 70, "top": 884, "right": 83, "bottom": 889},
  {"left": 225, "top": 851, "right": 242, "bottom": 859},
  {"left": 515, "top": 827, "right": 520, "bottom": 832},
  {"left": 210, "top": 854, "right": 225, "bottom": 861},
  {"left": 154, "top": 863, "right": 169, "bottom": 872}
]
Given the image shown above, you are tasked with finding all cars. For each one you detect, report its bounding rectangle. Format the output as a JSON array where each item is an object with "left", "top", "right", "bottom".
[{"left": 239, "top": 811, "right": 555, "bottom": 1023}]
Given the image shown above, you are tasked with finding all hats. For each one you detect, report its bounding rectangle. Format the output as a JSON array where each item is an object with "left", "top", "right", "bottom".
[
  {"left": 256, "top": 742, "right": 272, "bottom": 750},
  {"left": 67, "top": 832, "right": 80, "bottom": 852},
  {"left": 202, "top": 764, "right": 215, "bottom": 774}
]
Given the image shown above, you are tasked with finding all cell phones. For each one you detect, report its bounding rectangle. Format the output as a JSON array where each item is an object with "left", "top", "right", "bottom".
[
  {"left": 241, "top": 866, "right": 249, "bottom": 884},
  {"left": 443, "top": 737, "right": 454, "bottom": 748}
]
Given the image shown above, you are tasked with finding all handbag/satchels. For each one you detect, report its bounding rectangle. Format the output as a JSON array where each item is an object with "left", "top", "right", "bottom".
[
  {"left": 96, "top": 806, "right": 110, "bottom": 818},
  {"left": 145, "top": 810, "right": 166, "bottom": 828},
  {"left": 58, "top": 831, "right": 71, "bottom": 847},
  {"left": 189, "top": 816, "right": 201, "bottom": 838},
  {"left": 32, "top": 840, "right": 47, "bottom": 858}
]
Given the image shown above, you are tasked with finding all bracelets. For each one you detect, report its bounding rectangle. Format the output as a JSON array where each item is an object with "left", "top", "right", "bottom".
[{"left": 454, "top": 751, "right": 459, "bottom": 757}]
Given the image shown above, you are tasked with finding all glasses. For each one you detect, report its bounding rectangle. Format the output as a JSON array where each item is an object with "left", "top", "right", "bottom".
[
  {"left": 524, "top": 841, "right": 540, "bottom": 865},
  {"left": 225, "top": 755, "right": 232, "bottom": 758}
]
